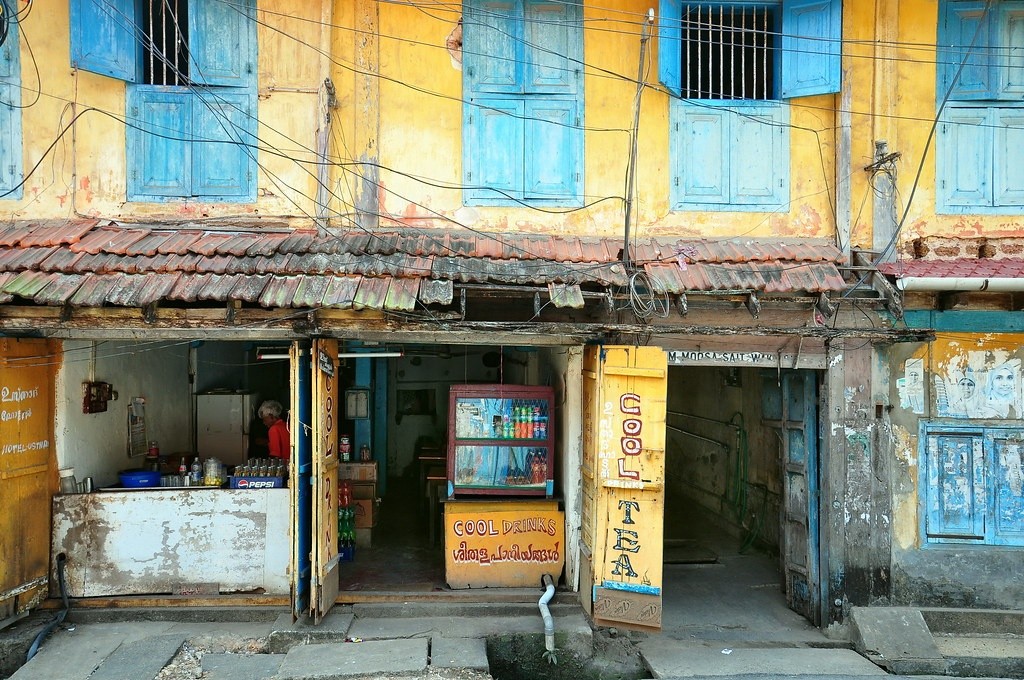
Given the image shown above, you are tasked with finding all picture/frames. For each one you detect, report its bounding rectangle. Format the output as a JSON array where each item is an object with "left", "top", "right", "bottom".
[{"left": 345, "top": 388, "right": 370, "bottom": 421}]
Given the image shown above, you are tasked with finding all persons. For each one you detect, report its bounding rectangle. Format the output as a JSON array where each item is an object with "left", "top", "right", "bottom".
[{"left": 259, "top": 400, "right": 290, "bottom": 460}]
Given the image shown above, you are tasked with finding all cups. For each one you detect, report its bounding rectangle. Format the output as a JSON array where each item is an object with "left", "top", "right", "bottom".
[{"left": 184, "top": 475, "right": 189, "bottom": 487}]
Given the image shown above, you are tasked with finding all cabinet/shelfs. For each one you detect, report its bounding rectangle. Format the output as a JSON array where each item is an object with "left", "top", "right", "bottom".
[
  {"left": 446, "top": 383, "right": 556, "bottom": 500},
  {"left": 338, "top": 459, "right": 378, "bottom": 483}
]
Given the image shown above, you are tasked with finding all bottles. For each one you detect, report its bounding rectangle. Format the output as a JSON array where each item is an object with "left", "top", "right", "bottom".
[
  {"left": 507, "top": 452, "right": 547, "bottom": 484},
  {"left": 360, "top": 444, "right": 370, "bottom": 461},
  {"left": 340, "top": 435, "right": 350, "bottom": 462},
  {"left": 234, "top": 457, "right": 289, "bottom": 477},
  {"left": 503, "top": 403, "right": 547, "bottom": 439},
  {"left": 179, "top": 457, "right": 187, "bottom": 486},
  {"left": 59, "top": 467, "right": 78, "bottom": 494},
  {"left": 338, "top": 481, "right": 356, "bottom": 549},
  {"left": 161, "top": 475, "right": 180, "bottom": 487},
  {"left": 191, "top": 457, "right": 202, "bottom": 486}
]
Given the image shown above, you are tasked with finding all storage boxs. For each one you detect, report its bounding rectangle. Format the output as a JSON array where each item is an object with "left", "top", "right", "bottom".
[
  {"left": 353, "top": 498, "right": 378, "bottom": 528},
  {"left": 231, "top": 474, "right": 284, "bottom": 488}
]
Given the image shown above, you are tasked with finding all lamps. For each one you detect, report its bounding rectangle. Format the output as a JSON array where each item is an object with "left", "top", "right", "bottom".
[
  {"left": 255, "top": 342, "right": 404, "bottom": 359},
  {"left": 724, "top": 367, "right": 742, "bottom": 388}
]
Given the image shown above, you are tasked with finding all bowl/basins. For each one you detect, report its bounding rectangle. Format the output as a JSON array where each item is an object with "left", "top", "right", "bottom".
[{"left": 119, "top": 471, "right": 161, "bottom": 487}]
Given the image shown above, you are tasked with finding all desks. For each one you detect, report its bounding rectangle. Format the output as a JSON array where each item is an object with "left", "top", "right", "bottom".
[{"left": 416, "top": 446, "right": 448, "bottom": 541}]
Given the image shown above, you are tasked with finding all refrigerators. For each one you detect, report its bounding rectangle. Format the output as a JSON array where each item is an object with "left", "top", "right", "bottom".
[{"left": 197, "top": 393, "right": 260, "bottom": 465}]
